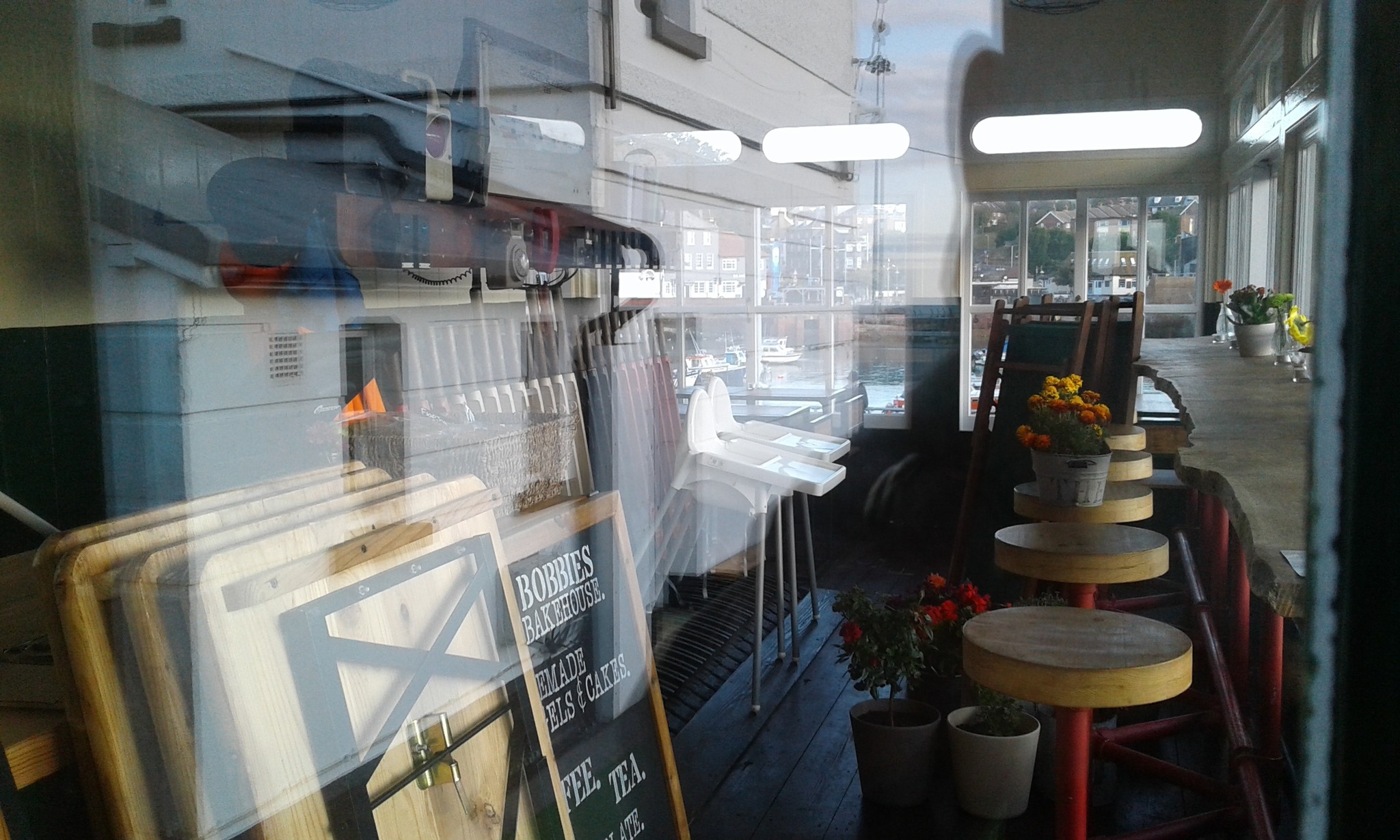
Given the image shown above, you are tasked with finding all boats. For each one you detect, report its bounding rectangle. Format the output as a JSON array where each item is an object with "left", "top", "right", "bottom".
[
  {"left": 717, "top": 329, "right": 746, "bottom": 366},
  {"left": 759, "top": 323, "right": 802, "bottom": 364},
  {"left": 880, "top": 344, "right": 1008, "bottom": 414},
  {"left": 669, "top": 328, "right": 747, "bottom": 391}
]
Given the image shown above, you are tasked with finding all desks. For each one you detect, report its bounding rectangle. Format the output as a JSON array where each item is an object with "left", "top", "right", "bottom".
[
  {"left": 678, "top": 404, "right": 809, "bottom": 430},
  {"left": 674, "top": 385, "right": 846, "bottom": 412},
  {"left": 0, "top": 459, "right": 576, "bottom": 840}
]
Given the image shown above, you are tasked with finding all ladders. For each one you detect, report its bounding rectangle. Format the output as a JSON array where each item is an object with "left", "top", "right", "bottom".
[{"left": 949, "top": 289, "right": 1149, "bottom": 590}]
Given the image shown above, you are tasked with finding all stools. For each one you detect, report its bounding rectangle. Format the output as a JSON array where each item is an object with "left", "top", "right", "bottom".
[{"left": 962, "top": 424, "right": 1192, "bottom": 839}]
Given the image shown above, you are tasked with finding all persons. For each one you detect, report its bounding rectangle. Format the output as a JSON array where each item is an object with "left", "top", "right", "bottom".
[{"left": 834, "top": 370, "right": 869, "bottom": 413}]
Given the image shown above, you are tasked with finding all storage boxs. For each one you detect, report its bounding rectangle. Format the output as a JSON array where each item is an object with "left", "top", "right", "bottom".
[{"left": 308, "top": 412, "right": 581, "bottom": 517}]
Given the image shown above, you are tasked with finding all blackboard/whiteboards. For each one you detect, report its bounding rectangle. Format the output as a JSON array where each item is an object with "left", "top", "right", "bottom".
[{"left": 500, "top": 490, "right": 700, "bottom": 839}]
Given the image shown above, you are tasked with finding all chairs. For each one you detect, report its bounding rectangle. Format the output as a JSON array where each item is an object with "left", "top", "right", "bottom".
[{"left": 633, "top": 378, "right": 850, "bottom": 711}]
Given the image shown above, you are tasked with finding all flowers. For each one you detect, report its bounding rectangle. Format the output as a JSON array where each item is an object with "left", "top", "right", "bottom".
[
  {"left": 1215, "top": 278, "right": 1231, "bottom": 293},
  {"left": 828, "top": 573, "right": 993, "bottom": 686},
  {"left": 1286, "top": 312, "right": 1311, "bottom": 342},
  {"left": 1237, "top": 286, "right": 1293, "bottom": 309},
  {"left": 1023, "top": 372, "right": 1108, "bottom": 445}
]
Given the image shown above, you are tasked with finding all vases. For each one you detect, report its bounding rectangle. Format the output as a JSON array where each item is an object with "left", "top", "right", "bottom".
[
  {"left": 1032, "top": 448, "right": 1111, "bottom": 505},
  {"left": 849, "top": 698, "right": 942, "bottom": 805},
  {"left": 948, "top": 706, "right": 1040, "bottom": 819},
  {"left": 1288, "top": 347, "right": 1311, "bottom": 382},
  {"left": 1234, "top": 323, "right": 1287, "bottom": 366},
  {"left": 1217, "top": 303, "right": 1235, "bottom": 337}
]
